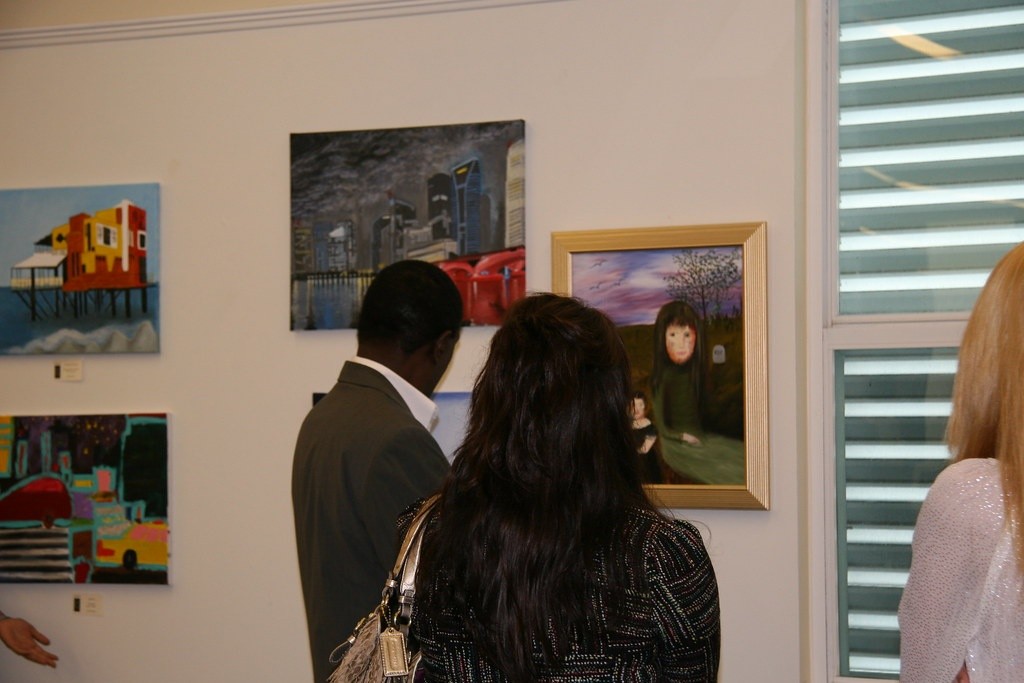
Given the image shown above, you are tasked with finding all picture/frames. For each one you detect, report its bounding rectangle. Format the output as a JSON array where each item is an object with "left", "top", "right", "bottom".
[{"left": 550, "top": 221, "right": 773, "bottom": 512}]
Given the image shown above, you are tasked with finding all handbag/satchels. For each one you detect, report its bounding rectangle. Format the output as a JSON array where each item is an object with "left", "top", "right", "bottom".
[{"left": 322, "top": 494, "right": 440, "bottom": 683}]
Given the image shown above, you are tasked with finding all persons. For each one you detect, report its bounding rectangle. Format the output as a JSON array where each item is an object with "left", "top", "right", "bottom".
[
  {"left": 382, "top": 295, "right": 722, "bottom": 683},
  {"left": 0, "top": 611, "right": 59, "bottom": 668},
  {"left": 292, "top": 260, "right": 454, "bottom": 683},
  {"left": 897, "top": 242, "right": 1024, "bottom": 683}
]
